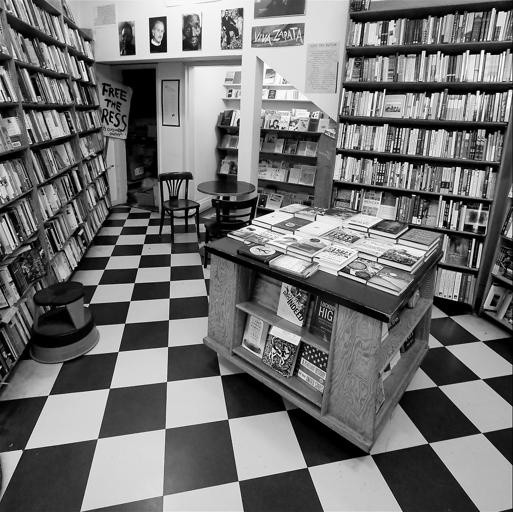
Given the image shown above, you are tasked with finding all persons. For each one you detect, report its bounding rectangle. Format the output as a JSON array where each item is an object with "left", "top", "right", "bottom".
[
  {"left": 222, "top": 10, "right": 240, "bottom": 49},
  {"left": 120, "top": 22, "right": 135, "bottom": 55},
  {"left": 150, "top": 20, "right": 166, "bottom": 52},
  {"left": 183, "top": 14, "right": 201, "bottom": 50}
]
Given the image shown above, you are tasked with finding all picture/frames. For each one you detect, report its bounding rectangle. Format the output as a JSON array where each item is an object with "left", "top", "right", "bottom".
[{"left": 160, "top": 79, "right": 180, "bottom": 127}]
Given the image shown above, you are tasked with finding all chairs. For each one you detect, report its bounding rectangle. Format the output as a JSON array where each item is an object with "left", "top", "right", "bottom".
[
  {"left": 158, "top": 172, "right": 201, "bottom": 239},
  {"left": 203, "top": 196, "right": 258, "bottom": 269}
]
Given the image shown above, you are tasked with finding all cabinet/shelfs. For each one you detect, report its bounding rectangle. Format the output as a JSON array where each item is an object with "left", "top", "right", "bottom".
[
  {"left": 202, "top": 203, "right": 442, "bottom": 453},
  {"left": 0, "top": 0, "right": 113, "bottom": 392},
  {"left": 478, "top": 184, "right": 513, "bottom": 331},
  {"left": 327, "top": 0, "right": 512, "bottom": 315},
  {"left": 216, "top": 83, "right": 336, "bottom": 212}
]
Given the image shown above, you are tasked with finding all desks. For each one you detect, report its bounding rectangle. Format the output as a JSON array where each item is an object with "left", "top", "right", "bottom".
[{"left": 196, "top": 180, "right": 255, "bottom": 259}]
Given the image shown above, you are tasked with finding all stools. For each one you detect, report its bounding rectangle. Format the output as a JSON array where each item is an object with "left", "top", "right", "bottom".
[{"left": 28, "top": 281, "right": 100, "bottom": 363}]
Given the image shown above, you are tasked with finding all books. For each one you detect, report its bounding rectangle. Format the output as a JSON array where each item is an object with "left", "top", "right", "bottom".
[
  {"left": 346, "top": 19, "right": 511, "bottom": 83},
  {"left": 0, "top": 1, "right": 104, "bottom": 206},
  {"left": 227, "top": 203, "right": 482, "bottom": 306},
  {"left": 221, "top": 109, "right": 330, "bottom": 211},
  {"left": 0, "top": 155, "right": 110, "bottom": 388},
  {"left": 329, "top": 154, "right": 499, "bottom": 237},
  {"left": 335, "top": 88, "right": 512, "bottom": 163},
  {"left": 239, "top": 273, "right": 335, "bottom": 394}
]
